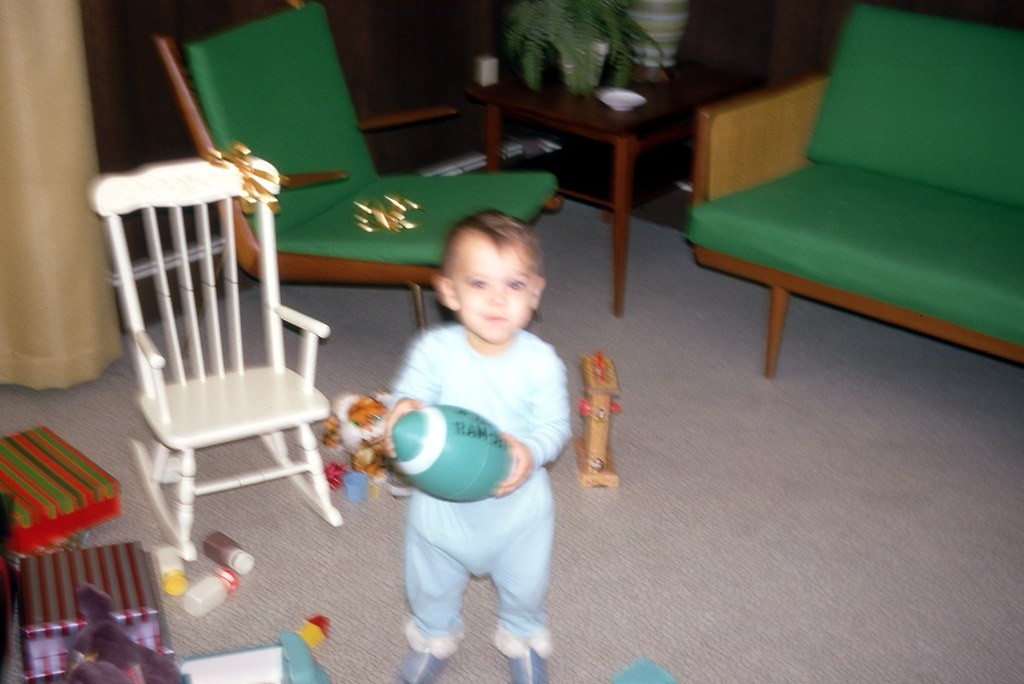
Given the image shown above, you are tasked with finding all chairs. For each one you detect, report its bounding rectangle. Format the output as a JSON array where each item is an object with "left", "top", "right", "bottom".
[
  {"left": 150, "top": 0, "right": 564, "bottom": 330},
  {"left": 89, "top": 157, "right": 345, "bottom": 562}
]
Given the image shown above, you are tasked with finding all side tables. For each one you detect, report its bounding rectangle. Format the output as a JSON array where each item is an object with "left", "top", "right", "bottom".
[{"left": 463, "top": 65, "right": 752, "bottom": 319}]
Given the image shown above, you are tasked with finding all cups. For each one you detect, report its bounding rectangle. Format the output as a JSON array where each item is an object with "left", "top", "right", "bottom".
[{"left": 341, "top": 474, "right": 369, "bottom": 503}]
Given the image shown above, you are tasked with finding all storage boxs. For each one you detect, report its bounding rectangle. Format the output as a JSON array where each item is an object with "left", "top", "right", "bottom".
[{"left": 0, "top": 426, "right": 174, "bottom": 683}]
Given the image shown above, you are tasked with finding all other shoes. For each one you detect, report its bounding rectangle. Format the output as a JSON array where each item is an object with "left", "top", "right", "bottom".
[
  {"left": 397, "top": 650, "right": 447, "bottom": 684},
  {"left": 509, "top": 649, "right": 547, "bottom": 684}
]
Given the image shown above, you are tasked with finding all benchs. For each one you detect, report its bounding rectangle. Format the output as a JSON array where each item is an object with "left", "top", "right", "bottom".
[{"left": 681, "top": 0, "right": 1024, "bottom": 377}]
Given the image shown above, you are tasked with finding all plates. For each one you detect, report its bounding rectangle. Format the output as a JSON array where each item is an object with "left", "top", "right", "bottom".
[{"left": 598, "top": 89, "right": 646, "bottom": 112}]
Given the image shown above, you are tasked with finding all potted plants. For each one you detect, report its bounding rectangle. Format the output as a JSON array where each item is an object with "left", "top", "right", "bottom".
[{"left": 500, "top": 1, "right": 667, "bottom": 96}]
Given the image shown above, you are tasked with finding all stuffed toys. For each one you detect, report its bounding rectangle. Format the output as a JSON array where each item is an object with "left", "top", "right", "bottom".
[{"left": 320, "top": 390, "right": 391, "bottom": 492}]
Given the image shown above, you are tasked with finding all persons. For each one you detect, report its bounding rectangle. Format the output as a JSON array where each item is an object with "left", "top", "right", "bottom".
[{"left": 382, "top": 208, "right": 573, "bottom": 684}]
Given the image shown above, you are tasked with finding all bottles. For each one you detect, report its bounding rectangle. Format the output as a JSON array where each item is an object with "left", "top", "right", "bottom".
[
  {"left": 182, "top": 568, "right": 238, "bottom": 618},
  {"left": 204, "top": 531, "right": 255, "bottom": 576},
  {"left": 156, "top": 546, "right": 189, "bottom": 597}
]
